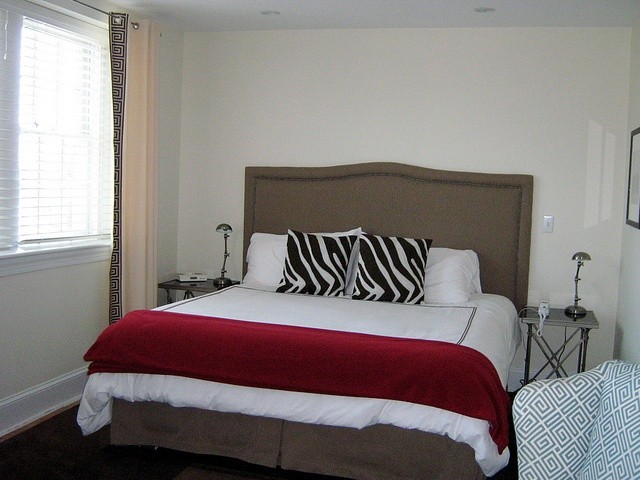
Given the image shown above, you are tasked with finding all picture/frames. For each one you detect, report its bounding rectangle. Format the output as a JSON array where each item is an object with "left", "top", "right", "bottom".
[{"left": 625, "top": 126, "right": 640, "bottom": 229}]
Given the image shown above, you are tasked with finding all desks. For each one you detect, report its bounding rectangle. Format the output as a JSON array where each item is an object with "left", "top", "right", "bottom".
[
  {"left": 158, "top": 278, "right": 241, "bottom": 305},
  {"left": 521, "top": 305, "right": 601, "bottom": 385}
]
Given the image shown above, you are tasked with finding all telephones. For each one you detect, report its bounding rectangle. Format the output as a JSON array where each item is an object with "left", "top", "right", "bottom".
[{"left": 536, "top": 300, "right": 549, "bottom": 337}]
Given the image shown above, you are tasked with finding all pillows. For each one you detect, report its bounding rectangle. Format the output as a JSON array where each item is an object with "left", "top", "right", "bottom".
[
  {"left": 276, "top": 228, "right": 358, "bottom": 297},
  {"left": 243, "top": 226, "right": 364, "bottom": 298},
  {"left": 347, "top": 242, "right": 481, "bottom": 307},
  {"left": 580, "top": 362, "right": 640, "bottom": 480},
  {"left": 352, "top": 232, "right": 433, "bottom": 304}
]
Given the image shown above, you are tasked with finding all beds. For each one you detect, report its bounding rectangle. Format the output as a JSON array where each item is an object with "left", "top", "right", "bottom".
[{"left": 74, "top": 160, "right": 536, "bottom": 478}]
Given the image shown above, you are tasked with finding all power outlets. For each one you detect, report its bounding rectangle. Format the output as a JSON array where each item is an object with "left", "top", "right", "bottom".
[{"left": 543, "top": 215, "right": 553, "bottom": 232}]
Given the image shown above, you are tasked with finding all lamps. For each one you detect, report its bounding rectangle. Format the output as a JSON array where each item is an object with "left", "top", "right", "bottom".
[
  {"left": 214, "top": 223, "right": 234, "bottom": 286},
  {"left": 565, "top": 252, "right": 592, "bottom": 315}
]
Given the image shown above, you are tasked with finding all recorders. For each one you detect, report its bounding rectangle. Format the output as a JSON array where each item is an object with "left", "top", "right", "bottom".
[{"left": 176, "top": 271, "right": 207, "bottom": 281}]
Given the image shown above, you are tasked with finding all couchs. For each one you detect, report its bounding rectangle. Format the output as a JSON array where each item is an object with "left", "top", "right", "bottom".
[{"left": 511, "top": 358, "right": 640, "bottom": 480}]
